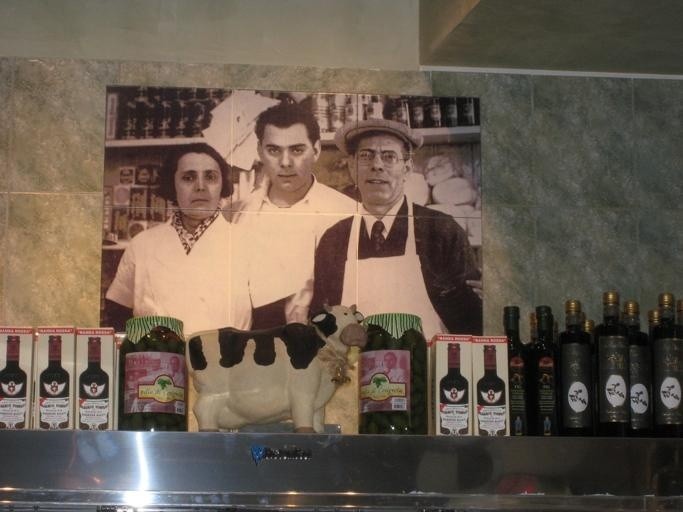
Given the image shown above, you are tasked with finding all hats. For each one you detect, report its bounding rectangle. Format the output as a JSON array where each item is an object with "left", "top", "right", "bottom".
[{"left": 334, "top": 118, "right": 425, "bottom": 156}]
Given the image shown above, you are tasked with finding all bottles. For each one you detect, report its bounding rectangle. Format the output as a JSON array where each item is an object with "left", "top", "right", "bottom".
[
  {"left": 118, "top": 86, "right": 476, "bottom": 141},
  {"left": 476, "top": 345, "right": 505, "bottom": 434},
  {"left": 0, "top": 336, "right": 31, "bottom": 428},
  {"left": 439, "top": 342, "right": 469, "bottom": 434},
  {"left": 78, "top": 332, "right": 110, "bottom": 428},
  {"left": 502, "top": 291, "right": 682, "bottom": 433},
  {"left": 38, "top": 336, "right": 72, "bottom": 428}
]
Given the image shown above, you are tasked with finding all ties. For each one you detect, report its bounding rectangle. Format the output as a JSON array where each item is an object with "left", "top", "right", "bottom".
[{"left": 370, "top": 221, "right": 386, "bottom": 249}]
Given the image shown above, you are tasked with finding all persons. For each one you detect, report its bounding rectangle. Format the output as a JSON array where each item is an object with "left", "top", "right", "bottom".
[
  {"left": 135, "top": 166, "right": 150, "bottom": 186},
  {"left": 309, "top": 121, "right": 482, "bottom": 345},
  {"left": 104, "top": 143, "right": 287, "bottom": 332},
  {"left": 117, "top": 164, "right": 136, "bottom": 186},
  {"left": 221, "top": 105, "right": 362, "bottom": 332},
  {"left": 465, "top": 267, "right": 483, "bottom": 300},
  {"left": 360, "top": 351, "right": 405, "bottom": 412},
  {"left": 129, "top": 354, "right": 183, "bottom": 413}
]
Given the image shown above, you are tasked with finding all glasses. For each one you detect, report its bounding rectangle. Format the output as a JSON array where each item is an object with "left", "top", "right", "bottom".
[{"left": 357, "top": 149, "right": 405, "bottom": 166}]
